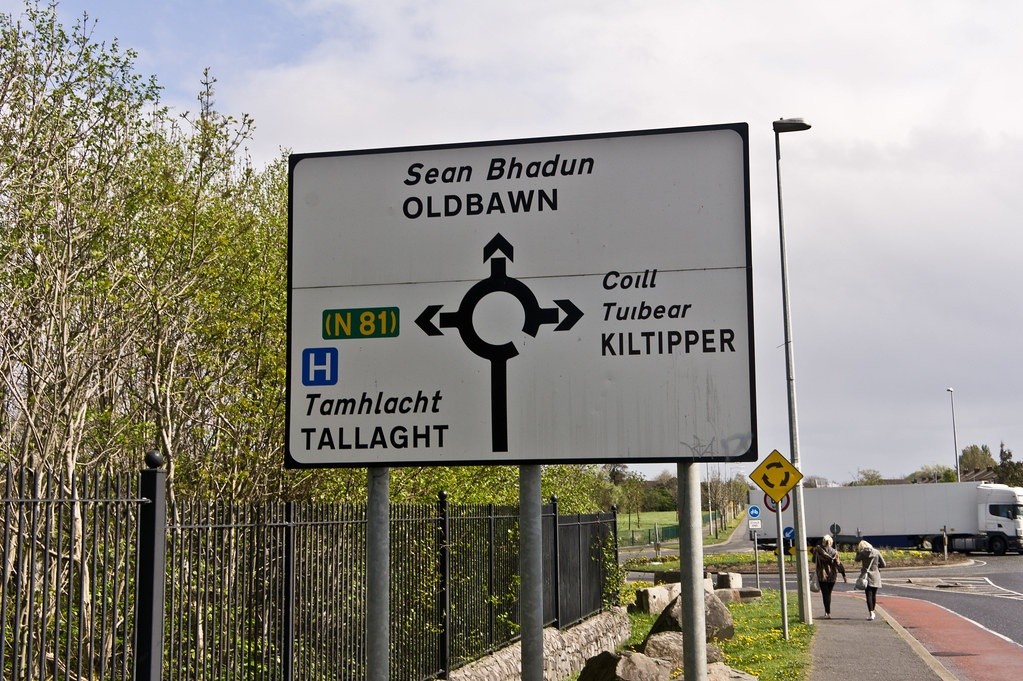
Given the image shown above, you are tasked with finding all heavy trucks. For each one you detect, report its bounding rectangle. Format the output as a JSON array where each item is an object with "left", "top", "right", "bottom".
[{"left": 748, "top": 481, "right": 1023, "bottom": 555}]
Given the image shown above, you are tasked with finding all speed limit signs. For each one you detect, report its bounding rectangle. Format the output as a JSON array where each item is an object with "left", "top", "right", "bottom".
[{"left": 764, "top": 491, "right": 791, "bottom": 512}]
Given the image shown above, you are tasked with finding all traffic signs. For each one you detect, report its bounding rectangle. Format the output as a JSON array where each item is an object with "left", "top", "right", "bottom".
[{"left": 283, "top": 123, "right": 759, "bottom": 469}]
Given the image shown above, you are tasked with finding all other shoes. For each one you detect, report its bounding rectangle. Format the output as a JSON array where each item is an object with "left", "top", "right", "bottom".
[
  {"left": 867, "top": 610, "right": 876, "bottom": 620},
  {"left": 824, "top": 612, "right": 831, "bottom": 619}
]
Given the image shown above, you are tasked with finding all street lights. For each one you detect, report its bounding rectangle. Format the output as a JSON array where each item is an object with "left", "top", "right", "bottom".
[
  {"left": 947, "top": 388, "right": 961, "bottom": 482},
  {"left": 772, "top": 117, "right": 883, "bottom": 531}
]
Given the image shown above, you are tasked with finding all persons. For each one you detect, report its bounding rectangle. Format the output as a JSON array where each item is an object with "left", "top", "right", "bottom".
[
  {"left": 812, "top": 535, "right": 847, "bottom": 619},
  {"left": 855, "top": 539, "right": 887, "bottom": 620}
]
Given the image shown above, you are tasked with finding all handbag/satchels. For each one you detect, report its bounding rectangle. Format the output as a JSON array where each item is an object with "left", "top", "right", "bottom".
[
  {"left": 856, "top": 578, "right": 868, "bottom": 590},
  {"left": 810, "top": 572, "right": 821, "bottom": 592}
]
